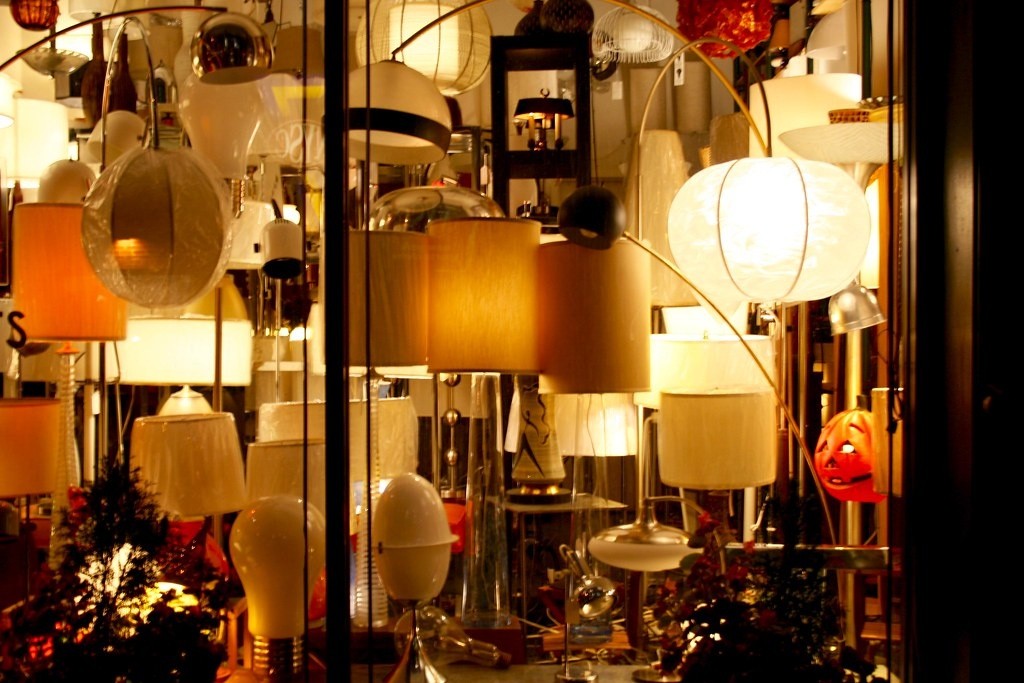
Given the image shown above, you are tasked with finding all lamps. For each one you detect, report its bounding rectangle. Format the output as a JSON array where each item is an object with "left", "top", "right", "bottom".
[
  {"left": 748, "top": 56, "right": 862, "bottom": 177},
  {"left": 354, "top": 0, "right": 493, "bottom": 97},
  {"left": 11, "top": 204, "right": 127, "bottom": 566},
  {"left": 0, "top": 399, "right": 59, "bottom": 610},
  {"left": 127, "top": 411, "right": 248, "bottom": 520},
  {"left": 260, "top": 396, "right": 419, "bottom": 478},
  {"left": 557, "top": 185, "right": 838, "bottom": 546},
  {"left": 0, "top": 5, "right": 275, "bottom": 85},
  {"left": 828, "top": 282, "right": 887, "bottom": 337},
  {"left": 502, "top": 376, "right": 643, "bottom": 535},
  {"left": 595, "top": 390, "right": 775, "bottom": 545},
  {"left": 511, "top": 85, "right": 574, "bottom": 225},
  {"left": 261, "top": 219, "right": 727, "bottom": 683},
  {"left": 591, "top": 0, "right": 673, "bottom": 64},
  {"left": 16, "top": 17, "right": 253, "bottom": 419},
  {"left": 320, "top": 0, "right": 451, "bottom": 166},
  {"left": 632, "top": 327, "right": 774, "bottom": 411},
  {"left": 244, "top": 440, "right": 360, "bottom": 541},
  {"left": 603, "top": 0, "right": 872, "bottom": 303}
]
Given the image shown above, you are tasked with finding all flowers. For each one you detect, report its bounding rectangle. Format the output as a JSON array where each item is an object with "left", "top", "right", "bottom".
[{"left": 643, "top": 511, "right": 850, "bottom": 683}]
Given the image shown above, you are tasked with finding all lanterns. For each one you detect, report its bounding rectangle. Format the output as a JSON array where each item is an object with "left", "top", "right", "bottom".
[
  {"left": 82, "top": 146, "right": 232, "bottom": 317},
  {"left": 10, "top": 1, "right": 58, "bottom": 30},
  {"left": 814, "top": 410, "right": 888, "bottom": 501},
  {"left": 669, "top": 157, "right": 872, "bottom": 300},
  {"left": 355, "top": 0, "right": 489, "bottom": 95}
]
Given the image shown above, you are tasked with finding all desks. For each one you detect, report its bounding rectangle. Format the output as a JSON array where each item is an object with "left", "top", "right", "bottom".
[{"left": 488, "top": 496, "right": 628, "bottom": 640}]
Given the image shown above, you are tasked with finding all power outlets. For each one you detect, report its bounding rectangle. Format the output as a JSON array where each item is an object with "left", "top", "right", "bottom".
[{"left": 673, "top": 49, "right": 685, "bottom": 87}]
